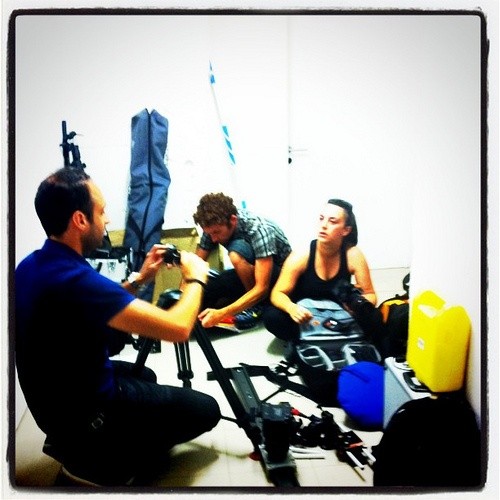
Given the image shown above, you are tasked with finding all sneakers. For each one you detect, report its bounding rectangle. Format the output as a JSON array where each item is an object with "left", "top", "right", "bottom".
[{"left": 233, "top": 302, "right": 264, "bottom": 329}]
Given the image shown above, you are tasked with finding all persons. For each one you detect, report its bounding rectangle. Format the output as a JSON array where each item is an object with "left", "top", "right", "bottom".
[
  {"left": 270, "top": 199, "right": 377, "bottom": 340},
  {"left": 191, "top": 193, "right": 292, "bottom": 328},
  {"left": 16, "top": 167, "right": 221, "bottom": 489}
]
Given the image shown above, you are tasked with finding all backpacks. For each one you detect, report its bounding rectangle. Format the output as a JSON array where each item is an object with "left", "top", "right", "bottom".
[
  {"left": 372, "top": 394, "right": 481, "bottom": 486},
  {"left": 376, "top": 273, "right": 411, "bottom": 363}
]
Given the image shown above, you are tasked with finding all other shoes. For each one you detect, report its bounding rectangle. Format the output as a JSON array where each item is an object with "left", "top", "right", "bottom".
[
  {"left": 43, "top": 437, "right": 64, "bottom": 463},
  {"left": 62, "top": 460, "right": 134, "bottom": 489},
  {"left": 283, "top": 341, "right": 300, "bottom": 362}
]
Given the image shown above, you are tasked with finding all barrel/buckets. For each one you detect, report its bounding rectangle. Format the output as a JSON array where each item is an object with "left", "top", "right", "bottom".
[{"left": 405, "top": 289, "right": 471, "bottom": 392}]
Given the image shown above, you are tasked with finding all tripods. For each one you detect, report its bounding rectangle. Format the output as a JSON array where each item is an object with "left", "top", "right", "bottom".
[{"left": 135, "top": 289, "right": 261, "bottom": 440}]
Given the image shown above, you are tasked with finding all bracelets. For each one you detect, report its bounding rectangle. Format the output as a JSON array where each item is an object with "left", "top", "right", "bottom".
[{"left": 186, "top": 277, "right": 208, "bottom": 290}]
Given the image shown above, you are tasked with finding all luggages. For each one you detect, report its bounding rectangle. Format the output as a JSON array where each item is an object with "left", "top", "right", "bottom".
[{"left": 294, "top": 297, "right": 382, "bottom": 393}]
